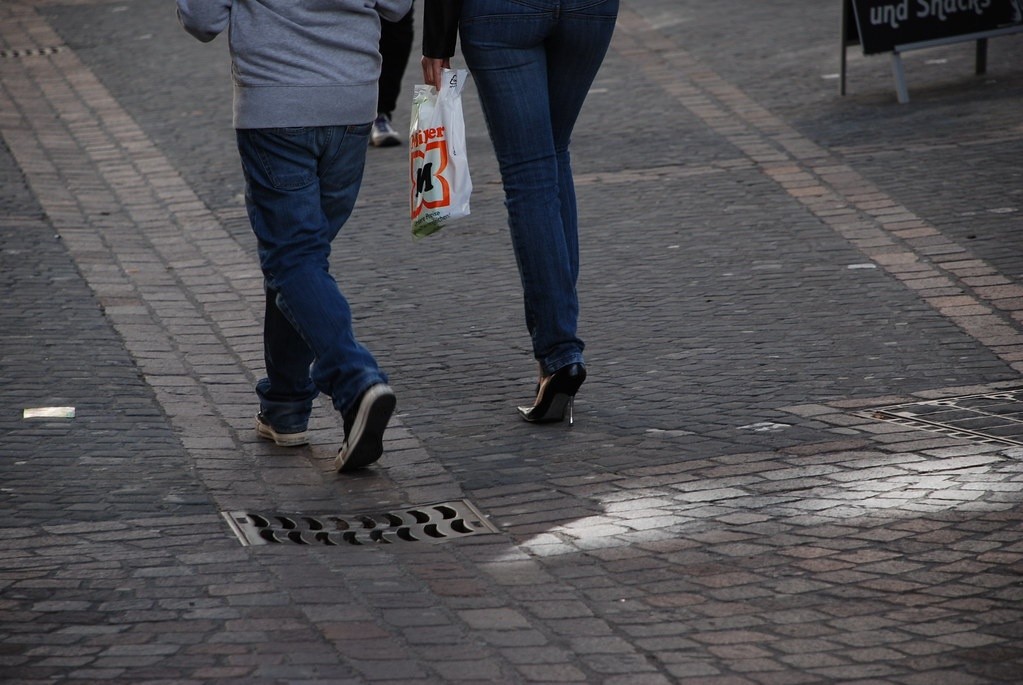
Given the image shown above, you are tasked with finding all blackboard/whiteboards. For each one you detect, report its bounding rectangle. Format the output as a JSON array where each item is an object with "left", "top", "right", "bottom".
[{"left": 843, "top": 0, "right": 1023, "bottom": 56}]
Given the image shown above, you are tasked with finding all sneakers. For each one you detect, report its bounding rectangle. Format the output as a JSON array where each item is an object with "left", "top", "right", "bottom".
[
  {"left": 334, "top": 383, "right": 397, "bottom": 474},
  {"left": 369, "top": 112, "right": 401, "bottom": 147},
  {"left": 253, "top": 412, "right": 311, "bottom": 448}
]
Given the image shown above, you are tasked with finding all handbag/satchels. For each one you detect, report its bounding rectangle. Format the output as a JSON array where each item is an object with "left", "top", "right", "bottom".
[{"left": 408, "top": 68, "right": 473, "bottom": 240}]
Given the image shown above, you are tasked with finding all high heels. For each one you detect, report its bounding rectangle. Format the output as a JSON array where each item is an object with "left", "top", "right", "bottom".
[{"left": 516, "top": 361, "right": 587, "bottom": 426}]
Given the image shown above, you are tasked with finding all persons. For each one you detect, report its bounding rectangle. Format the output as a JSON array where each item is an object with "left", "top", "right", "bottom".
[
  {"left": 176, "top": 0, "right": 415, "bottom": 474},
  {"left": 420, "top": 0, "right": 619, "bottom": 426}
]
